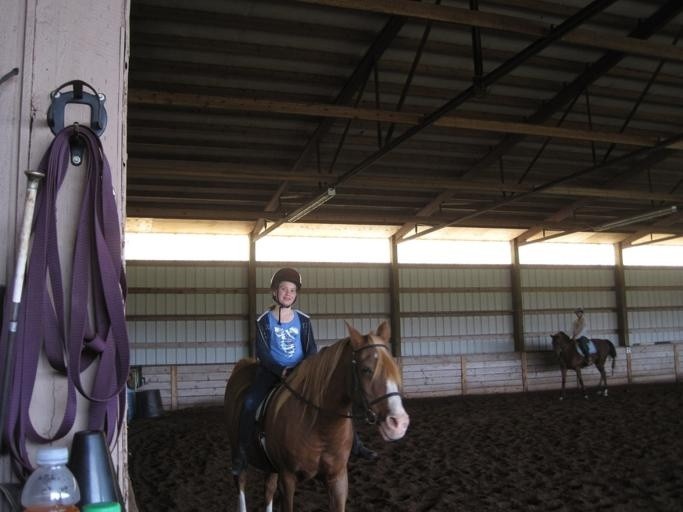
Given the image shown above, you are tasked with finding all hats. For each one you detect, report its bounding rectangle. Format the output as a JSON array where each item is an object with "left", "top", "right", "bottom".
[{"left": 574, "top": 307, "right": 583, "bottom": 313}]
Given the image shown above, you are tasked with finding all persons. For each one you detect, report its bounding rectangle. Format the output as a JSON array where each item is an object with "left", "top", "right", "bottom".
[
  {"left": 229, "top": 266, "right": 378, "bottom": 479},
  {"left": 568, "top": 304, "right": 593, "bottom": 366}
]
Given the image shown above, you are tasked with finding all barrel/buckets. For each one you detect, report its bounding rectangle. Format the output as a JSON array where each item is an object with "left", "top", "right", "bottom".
[{"left": 135, "top": 388, "right": 164, "bottom": 417}]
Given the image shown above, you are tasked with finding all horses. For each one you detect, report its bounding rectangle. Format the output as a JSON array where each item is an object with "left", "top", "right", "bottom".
[
  {"left": 549, "top": 330, "right": 617, "bottom": 400},
  {"left": 221, "top": 318, "right": 413, "bottom": 512}
]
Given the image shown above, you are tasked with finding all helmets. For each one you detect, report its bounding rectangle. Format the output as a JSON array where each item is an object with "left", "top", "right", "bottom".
[{"left": 270, "top": 268, "right": 301, "bottom": 290}]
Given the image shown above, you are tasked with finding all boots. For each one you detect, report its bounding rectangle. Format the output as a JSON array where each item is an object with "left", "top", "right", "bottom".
[
  {"left": 357, "top": 441, "right": 377, "bottom": 458},
  {"left": 231, "top": 406, "right": 252, "bottom": 476}
]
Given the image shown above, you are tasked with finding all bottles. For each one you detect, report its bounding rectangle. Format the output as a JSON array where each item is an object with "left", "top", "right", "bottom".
[{"left": 21, "top": 448, "right": 81, "bottom": 511}]
